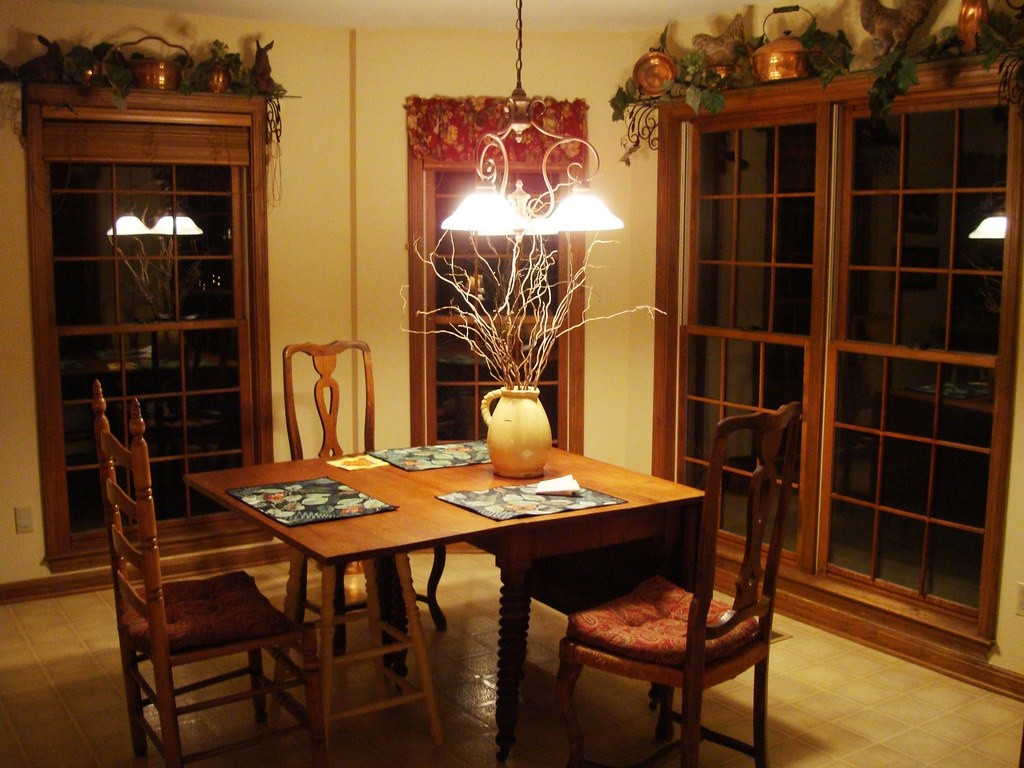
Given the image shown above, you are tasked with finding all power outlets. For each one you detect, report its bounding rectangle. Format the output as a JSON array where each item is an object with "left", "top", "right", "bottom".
[{"left": 14, "top": 504, "right": 35, "bottom": 535}]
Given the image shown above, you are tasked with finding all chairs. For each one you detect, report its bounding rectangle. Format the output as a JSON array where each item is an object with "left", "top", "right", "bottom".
[
  {"left": 278, "top": 340, "right": 446, "bottom": 653},
  {"left": 554, "top": 401, "right": 802, "bottom": 768},
  {"left": 92, "top": 379, "right": 326, "bottom": 768},
  {"left": 138, "top": 313, "right": 239, "bottom": 484}
]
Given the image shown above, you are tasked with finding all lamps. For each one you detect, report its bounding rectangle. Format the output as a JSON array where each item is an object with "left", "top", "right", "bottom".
[
  {"left": 968, "top": 183, "right": 1007, "bottom": 239},
  {"left": 441, "top": 1, "right": 624, "bottom": 235},
  {"left": 107, "top": 177, "right": 203, "bottom": 236}
]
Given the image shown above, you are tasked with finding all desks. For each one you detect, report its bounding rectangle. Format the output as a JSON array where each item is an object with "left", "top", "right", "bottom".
[
  {"left": 184, "top": 437, "right": 707, "bottom": 759},
  {"left": 59, "top": 345, "right": 239, "bottom": 492}
]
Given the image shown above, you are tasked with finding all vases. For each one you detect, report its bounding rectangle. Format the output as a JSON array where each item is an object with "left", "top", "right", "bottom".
[{"left": 482, "top": 385, "right": 551, "bottom": 477}]
